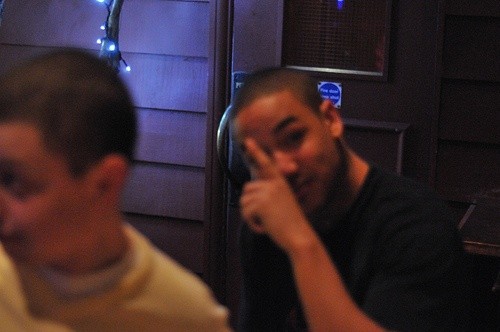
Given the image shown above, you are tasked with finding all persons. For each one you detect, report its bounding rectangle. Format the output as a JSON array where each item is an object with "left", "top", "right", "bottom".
[
  {"left": 0, "top": 45, "right": 231, "bottom": 332},
  {"left": 231, "top": 67, "right": 485, "bottom": 332}
]
